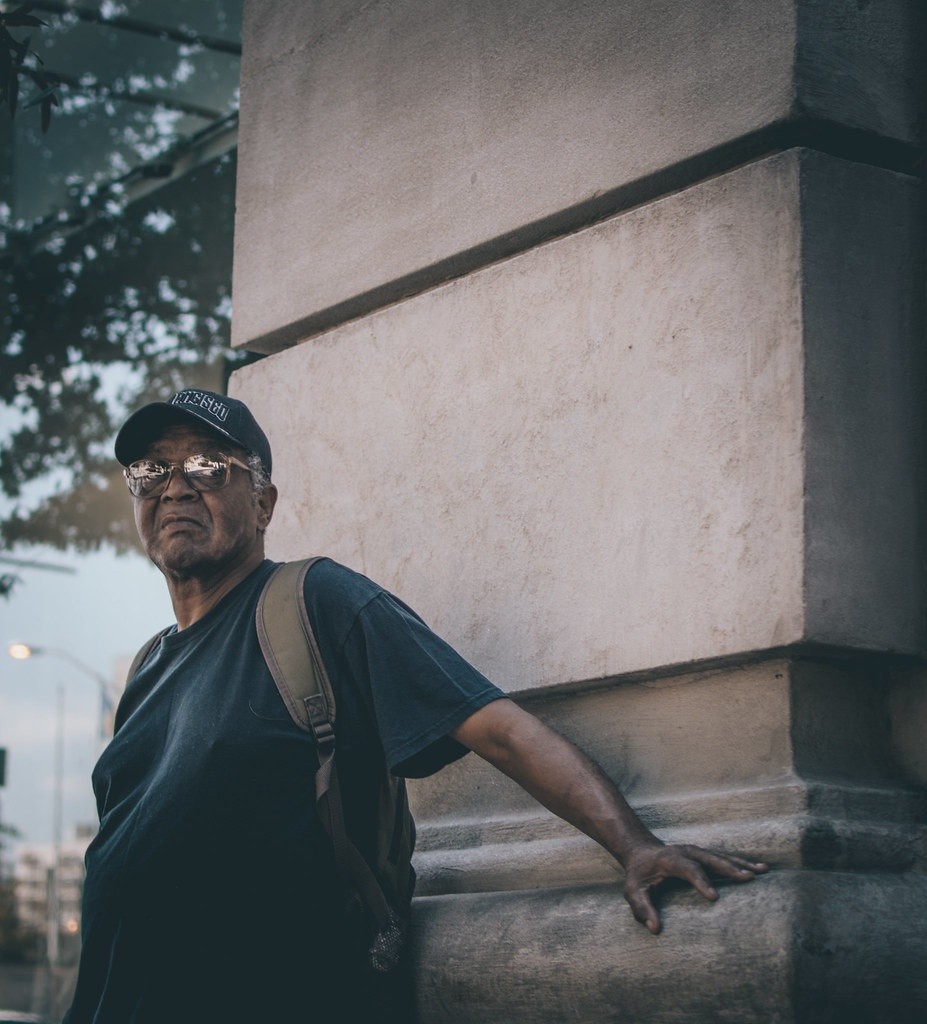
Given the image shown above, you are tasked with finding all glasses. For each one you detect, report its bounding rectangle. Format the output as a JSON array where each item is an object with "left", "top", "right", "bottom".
[{"left": 122, "top": 452, "right": 263, "bottom": 499}]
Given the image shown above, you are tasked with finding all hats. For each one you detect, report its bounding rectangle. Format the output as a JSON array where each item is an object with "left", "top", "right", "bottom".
[{"left": 115, "top": 387, "right": 272, "bottom": 482}]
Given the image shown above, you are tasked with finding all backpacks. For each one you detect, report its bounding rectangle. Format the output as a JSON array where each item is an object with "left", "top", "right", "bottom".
[{"left": 259, "top": 558, "right": 417, "bottom": 1017}]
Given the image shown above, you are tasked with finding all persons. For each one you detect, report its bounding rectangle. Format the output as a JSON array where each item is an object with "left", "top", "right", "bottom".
[{"left": 54, "top": 387, "right": 771, "bottom": 1024}]
